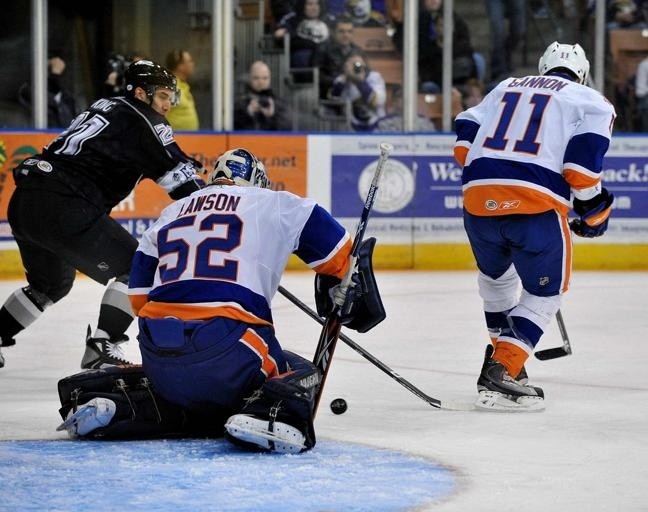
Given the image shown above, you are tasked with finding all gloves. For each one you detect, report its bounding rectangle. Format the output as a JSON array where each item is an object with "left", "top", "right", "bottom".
[
  {"left": 328, "top": 254, "right": 364, "bottom": 317},
  {"left": 568, "top": 186, "right": 615, "bottom": 238}
]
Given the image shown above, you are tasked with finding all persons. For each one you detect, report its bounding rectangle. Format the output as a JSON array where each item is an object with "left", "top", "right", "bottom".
[
  {"left": 453, "top": 42, "right": 617, "bottom": 411},
  {"left": 56, "top": 147, "right": 370, "bottom": 455},
  {"left": 0, "top": 60, "right": 211, "bottom": 373}
]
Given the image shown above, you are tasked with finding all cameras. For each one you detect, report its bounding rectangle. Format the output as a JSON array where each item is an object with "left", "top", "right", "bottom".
[
  {"left": 259, "top": 89, "right": 273, "bottom": 107},
  {"left": 353, "top": 63, "right": 362, "bottom": 73}
]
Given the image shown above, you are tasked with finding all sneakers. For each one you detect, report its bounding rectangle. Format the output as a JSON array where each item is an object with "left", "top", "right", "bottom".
[
  {"left": 224, "top": 413, "right": 306, "bottom": 456},
  {"left": 476, "top": 343, "right": 546, "bottom": 403},
  {"left": 80, "top": 323, "right": 144, "bottom": 373},
  {"left": 66, "top": 396, "right": 118, "bottom": 440}
]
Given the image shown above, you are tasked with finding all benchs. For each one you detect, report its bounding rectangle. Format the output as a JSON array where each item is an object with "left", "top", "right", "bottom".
[{"left": 234, "top": 1, "right": 648, "bottom": 133}]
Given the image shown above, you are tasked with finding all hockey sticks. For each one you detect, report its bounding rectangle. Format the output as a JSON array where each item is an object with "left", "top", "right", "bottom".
[
  {"left": 277, "top": 284, "right": 476, "bottom": 411},
  {"left": 535, "top": 308, "right": 572, "bottom": 361}
]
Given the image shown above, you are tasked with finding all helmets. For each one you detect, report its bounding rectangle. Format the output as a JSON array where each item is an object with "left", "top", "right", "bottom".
[
  {"left": 538, "top": 40, "right": 592, "bottom": 87},
  {"left": 207, "top": 147, "right": 271, "bottom": 189},
  {"left": 123, "top": 58, "right": 178, "bottom": 103}
]
